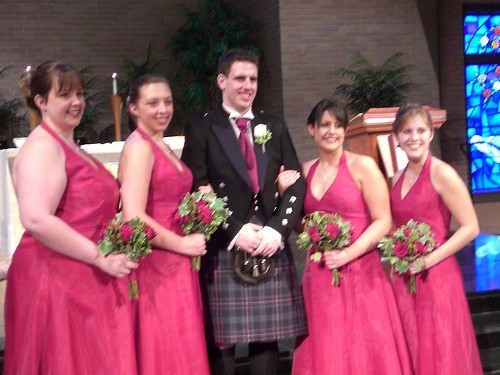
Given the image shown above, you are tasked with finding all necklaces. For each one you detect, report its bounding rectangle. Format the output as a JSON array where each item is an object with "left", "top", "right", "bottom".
[
  {"left": 314, "top": 155, "right": 340, "bottom": 197},
  {"left": 70, "top": 141, "right": 80, "bottom": 150}
]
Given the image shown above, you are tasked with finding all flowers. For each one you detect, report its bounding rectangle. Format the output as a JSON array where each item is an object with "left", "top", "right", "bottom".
[
  {"left": 376, "top": 216, "right": 439, "bottom": 296},
  {"left": 172, "top": 182, "right": 233, "bottom": 272},
  {"left": 295, "top": 211, "right": 356, "bottom": 288},
  {"left": 254, "top": 123, "right": 273, "bottom": 154},
  {"left": 98, "top": 218, "right": 158, "bottom": 302}
]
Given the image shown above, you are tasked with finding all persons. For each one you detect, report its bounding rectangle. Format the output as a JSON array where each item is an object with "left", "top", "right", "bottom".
[
  {"left": 183, "top": 48, "right": 307, "bottom": 375},
  {"left": 4, "top": 61, "right": 137, "bottom": 375},
  {"left": 118, "top": 72, "right": 214, "bottom": 375},
  {"left": 388, "top": 104, "right": 484, "bottom": 375},
  {"left": 277, "top": 98, "right": 413, "bottom": 375}
]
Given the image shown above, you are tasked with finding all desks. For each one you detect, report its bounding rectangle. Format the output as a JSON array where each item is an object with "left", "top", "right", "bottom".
[{"left": 0, "top": 137, "right": 187, "bottom": 355}]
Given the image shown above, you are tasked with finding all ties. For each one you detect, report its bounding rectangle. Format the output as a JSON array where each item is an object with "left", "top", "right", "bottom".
[{"left": 235, "top": 118, "right": 260, "bottom": 194}]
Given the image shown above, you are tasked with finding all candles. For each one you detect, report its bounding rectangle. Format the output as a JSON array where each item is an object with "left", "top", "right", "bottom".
[
  {"left": 112, "top": 73, "right": 117, "bottom": 95},
  {"left": 26, "top": 64, "right": 33, "bottom": 97}
]
